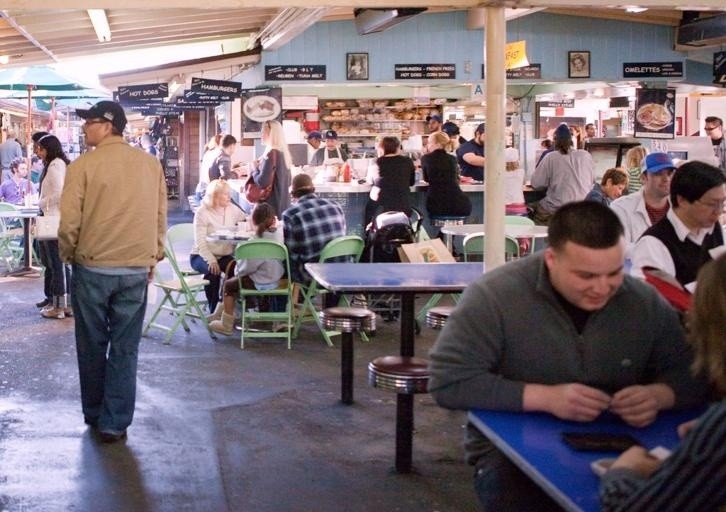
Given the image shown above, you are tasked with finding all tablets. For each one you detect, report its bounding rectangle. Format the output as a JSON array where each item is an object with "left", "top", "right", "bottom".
[{"left": 563, "top": 432, "right": 642, "bottom": 451}]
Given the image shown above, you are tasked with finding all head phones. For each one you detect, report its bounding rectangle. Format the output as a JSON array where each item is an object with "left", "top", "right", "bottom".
[{"left": 297, "top": 188, "right": 314, "bottom": 199}]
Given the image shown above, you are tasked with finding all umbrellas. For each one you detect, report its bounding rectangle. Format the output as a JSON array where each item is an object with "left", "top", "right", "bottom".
[{"left": 0, "top": 67, "right": 103, "bottom": 197}]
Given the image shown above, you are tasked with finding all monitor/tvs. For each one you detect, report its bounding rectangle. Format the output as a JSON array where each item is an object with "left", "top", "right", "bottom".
[{"left": 288, "top": 144, "right": 308, "bottom": 167}]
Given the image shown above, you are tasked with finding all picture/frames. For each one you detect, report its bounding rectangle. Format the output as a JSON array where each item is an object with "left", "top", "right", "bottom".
[
  {"left": 346, "top": 51, "right": 369, "bottom": 81},
  {"left": 567, "top": 50, "right": 591, "bottom": 80}
]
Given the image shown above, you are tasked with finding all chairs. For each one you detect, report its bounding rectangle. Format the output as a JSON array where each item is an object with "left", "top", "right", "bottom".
[
  {"left": 289, "top": 235, "right": 369, "bottom": 348},
  {"left": 141, "top": 245, "right": 221, "bottom": 347},
  {"left": 411, "top": 228, "right": 464, "bottom": 320},
  {"left": 464, "top": 234, "right": 522, "bottom": 262},
  {"left": 1, "top": 201, "right": 40, "bottom": 271},
  {"left": 167, "top": 221, "right": 214, "bottom": 324},
  {"left": 234, "top": 242, "right": 292, "bottom": 349},
  {"left": 507, "top": 215, "right": 535, "bottom": 255}
]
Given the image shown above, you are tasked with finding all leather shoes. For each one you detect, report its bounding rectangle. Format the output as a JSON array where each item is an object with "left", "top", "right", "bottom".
[{"left": 36, "top": 298, "right": 73, "bottom": 319}]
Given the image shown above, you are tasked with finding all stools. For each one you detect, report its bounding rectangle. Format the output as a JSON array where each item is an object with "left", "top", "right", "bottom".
[
  {"left": 317, "top": 306, "right": 376, "bottom": 403},
  {"left": 427, "top": 308, "right": 459, "bottom": 328},
  {"left": 367, "top": 355, "right": 433, "bottom": 473}
]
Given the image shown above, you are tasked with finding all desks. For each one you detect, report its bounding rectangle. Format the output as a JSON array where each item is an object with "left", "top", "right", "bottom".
[
  {"left": 440, "top": 222, "right": 548, "bottom": 263},
  {"left": 1, "top": 209, "right": 46, "bottom": 276},
  {"left": 468, "top": 394, "right": 725, "bottom": 512}
]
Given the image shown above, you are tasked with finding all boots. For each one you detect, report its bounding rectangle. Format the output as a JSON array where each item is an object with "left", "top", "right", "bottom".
[
  {"left": 208, "top": 311, "right": 235, "bottom": 336},
  {"left": 204, "top": 302, "right": 223, "bottom": 323}
]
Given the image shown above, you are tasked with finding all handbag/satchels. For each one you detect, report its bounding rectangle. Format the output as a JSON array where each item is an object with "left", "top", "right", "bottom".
[{"left": 244, "top": 168, "right": 274, "bottom": 202}]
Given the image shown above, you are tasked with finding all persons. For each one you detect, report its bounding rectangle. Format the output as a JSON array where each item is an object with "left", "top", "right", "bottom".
[
  {"left": 57, "top": 100, "right": 169, "bottom": 443},
  {"left": 193, "top": 111, "right": 529, "bottom": 337},
  {"left": 347, "top": 57, "right": 367, "bottom": 79},
  {"left": 0, "top": 129, "right": 74, "bottom": 319},
  {"left": 531, "top": 111, "right": 726, "bottom": 334},
  {"left": 572, "top": 55, "right": 587, "bottom": 75},
  {"left": 136, "top": 135, "right": 160, "bottom": 156},
  {"left": 598, "top": 253, "right": 726, "bottom": 512},
  {"left": 423, "top": 200, "right": 690, "bottom": 511}
]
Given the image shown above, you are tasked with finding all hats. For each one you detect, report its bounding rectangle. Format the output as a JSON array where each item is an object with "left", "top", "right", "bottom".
[
  {"left": 75, "top": 101, "right": 126, "bottom": 132},
  {"left": 291, "top": 115, "right": 459, "bottom": 192},
  {"left": 640, "top": 152, "right": 676, "bottom": 173}
]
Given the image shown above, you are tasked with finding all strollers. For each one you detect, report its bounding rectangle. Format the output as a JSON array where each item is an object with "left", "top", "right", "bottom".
[{"left": 349, "top": 204, "right": 426, "bottom": 338}]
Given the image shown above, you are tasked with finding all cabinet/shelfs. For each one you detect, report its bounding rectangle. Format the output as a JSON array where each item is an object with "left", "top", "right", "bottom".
[{"left": 319, "top": 100, "right": 444, "bottom": 141}]
